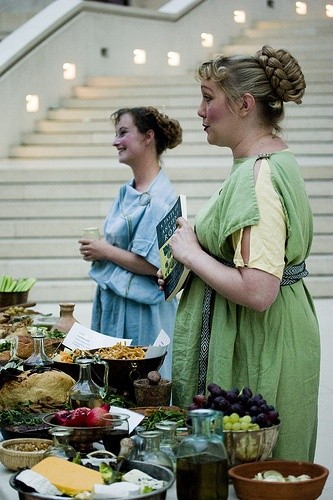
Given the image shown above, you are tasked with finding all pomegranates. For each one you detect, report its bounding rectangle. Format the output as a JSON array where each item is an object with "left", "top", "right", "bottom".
[{"left": 55, "top": 403, "right": 113, "bottom": 427}]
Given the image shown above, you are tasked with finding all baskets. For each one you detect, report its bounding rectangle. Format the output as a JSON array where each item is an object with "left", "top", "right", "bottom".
[{"left": 0, "top": 438, "right": 59, "bottom": 472}]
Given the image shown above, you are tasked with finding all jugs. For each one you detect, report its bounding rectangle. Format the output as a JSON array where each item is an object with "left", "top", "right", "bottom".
[
  {"left": 176, "top": 409, "right": 229, "bottom": 500},
  {"left": 69, "top": 359, "right": 109, "bottom": 410}
]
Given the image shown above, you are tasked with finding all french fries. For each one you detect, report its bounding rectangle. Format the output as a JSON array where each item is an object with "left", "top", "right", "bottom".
[
  {"left": 97, "top": 341, "right": 147, "bottom": 360},
  {"left": 59, "top": 350, "right": 74, "bottom": 363}
]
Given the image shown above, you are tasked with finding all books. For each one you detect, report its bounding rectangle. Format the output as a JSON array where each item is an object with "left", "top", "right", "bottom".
[{"left": 155, "top": 195, "right": 191, "bottom": 301}]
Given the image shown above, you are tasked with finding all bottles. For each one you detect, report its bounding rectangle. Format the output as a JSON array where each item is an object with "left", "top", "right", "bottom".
[
  {"left": 50, "top": 303, "right": 80, "bottom": 336},
  {"left": 23, "top": 335, "right": 55, "bottom": 376},
  {"left": 43, "top": 426, "right": 77, "bottom": 462},
  {"left": 135, "top": 422, "right": 180, "bottom": 475}
]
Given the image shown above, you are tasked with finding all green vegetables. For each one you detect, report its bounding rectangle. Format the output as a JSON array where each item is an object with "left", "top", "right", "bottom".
[
  {"left": 27, "top": 326, "right": 66, "bottom": 338},
  {"left": 0, "top": 400, "right": 45, "bottom": 426},
  {"left": 104, "top": 391, "right": 135, "bottom": 410},
  {"left": 138, "top": 406, "right": 187, "bottom": 430},
  {"left": 0, "top": 340, "right": 12, "bottom": 352}
]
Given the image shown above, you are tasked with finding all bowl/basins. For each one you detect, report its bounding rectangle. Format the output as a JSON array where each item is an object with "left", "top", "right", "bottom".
[
  {"left": 53, "top": 345, "right": 167, "bottom": 389},
  {"left": 0, "top": 289, "right": 31, "bottom": 313},
  {"left": 8, "top": 458, "right": 175, "bottom": 500},
  {"left": 185, "top": 417, "right": 281, "bottom": 485},
  {"left": 0, "top": 414, "right": 55, "bottom": 441},
  {"left": 228, "top": 460, "right": 329, "bottom": 500},
  {"left": 42, "top": 412, "right": 122, "bottom": 458},
  {"left": 0, "top": 438, "right": 58, "bottom": 471}
]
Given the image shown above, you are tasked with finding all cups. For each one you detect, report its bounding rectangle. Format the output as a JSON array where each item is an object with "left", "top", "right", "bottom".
[
  {"left": 83, "top": 228, "right": 100, "bottom": 241},
  {"left": 101, "top": 413, "right": 130, "bottom": 459}
]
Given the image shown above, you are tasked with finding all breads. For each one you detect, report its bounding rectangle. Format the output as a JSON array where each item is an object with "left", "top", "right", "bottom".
[
  {"left": 15, "top": 334, "right": 64, "bottom": 359},
  {"left": 0, "top": 366, "right": 76, "bottom": 409}
]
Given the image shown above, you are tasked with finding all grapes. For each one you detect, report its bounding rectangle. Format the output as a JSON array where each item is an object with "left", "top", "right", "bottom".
[{"left": 188, "top": 383, "right": 280, "bottom": 460}]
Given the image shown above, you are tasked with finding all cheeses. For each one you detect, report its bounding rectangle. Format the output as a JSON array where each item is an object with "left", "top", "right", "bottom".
[{"left": 27, "top": 456, "right": 105, "bottom": 495}]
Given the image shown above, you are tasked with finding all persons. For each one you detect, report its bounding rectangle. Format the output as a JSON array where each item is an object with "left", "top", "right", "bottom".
[
  {"left": 158, "top": 45, "right": 321, "bottom": 464},
  {"left": 76, "top": 107, "right": 182, "bottom": 383}
]
